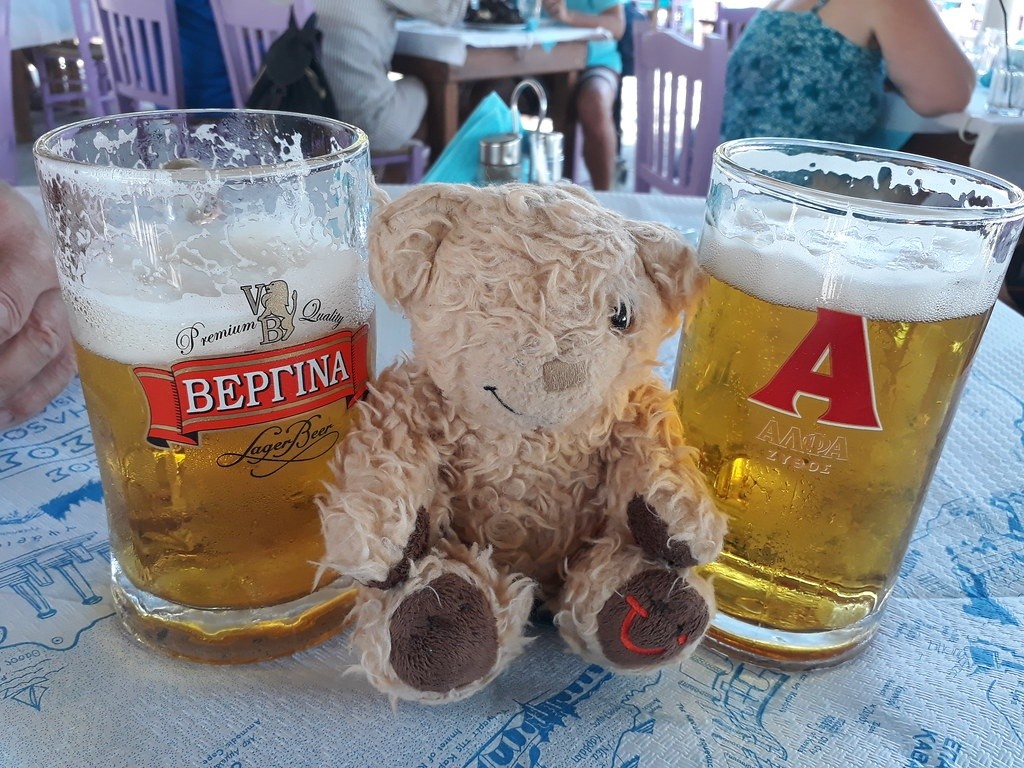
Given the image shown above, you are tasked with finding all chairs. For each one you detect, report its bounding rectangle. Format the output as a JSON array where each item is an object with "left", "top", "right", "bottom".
[
  {"left": 91, "top": 0, "right": 229, "bottom": 168},
  {"left": 715, "top": 3, "right": 758, "bottom": 51},
  {"left": 35, "top": 43, "right": 113, "bottom": 132},
  {"left": 210, "top": 0, "right": 432, "bottom": 185},
  {"left": 632, "top": 21, "right": 724, "bottom": 197}
]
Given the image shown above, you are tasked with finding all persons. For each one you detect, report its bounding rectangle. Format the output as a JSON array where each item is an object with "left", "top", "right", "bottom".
[
  {"left": 309, "top": 1, "right": 470, "bottom": 160},
  {"left": 483, "top": 0, "right": 627, "bottom": 189},
  {"left": 318, "top": 179, "right": 733, "bottom": 708},
  {"left": 719, "top": 0, "right": 976, "bottom": 206},
  {"left": 0, "top": 177, "right": 81, "bottom": 418}
]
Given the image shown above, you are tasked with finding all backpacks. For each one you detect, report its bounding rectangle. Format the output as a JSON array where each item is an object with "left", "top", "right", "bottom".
[{"left": 243, "top": 23, "right": 340, "bottom": 150}]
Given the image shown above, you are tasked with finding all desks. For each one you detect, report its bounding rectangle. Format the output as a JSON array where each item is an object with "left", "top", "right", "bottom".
[
  {"left": 0, "top": 184, "right": 1024, "bottom": 768},
  {"left": 871, "top": 85, "right": 1024, "bottom": 188},
  {"left": 393, "top": 20, "right": 613, "bottom": 182}
]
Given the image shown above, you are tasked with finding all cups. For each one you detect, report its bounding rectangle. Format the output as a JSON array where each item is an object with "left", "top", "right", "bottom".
[
  {"left": 672, "top": 136, "right": 1024, "bottom": 669},
  {"left": 516, "top": 0, "right": 541, "bottom": 22},
  {"left": 984, "top": 45, "right": 1024, "bottom": 118},
  {"left": 32, "top": 110, "right": 378, "bottom": 666}
]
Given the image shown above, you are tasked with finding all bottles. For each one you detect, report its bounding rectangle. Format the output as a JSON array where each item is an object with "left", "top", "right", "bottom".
[
  {"left": 531, "top": 132, "right": 564, "bottom": 185},
  {"left": 480, "top": 134, "right": 521, "bottom": 186}
]
